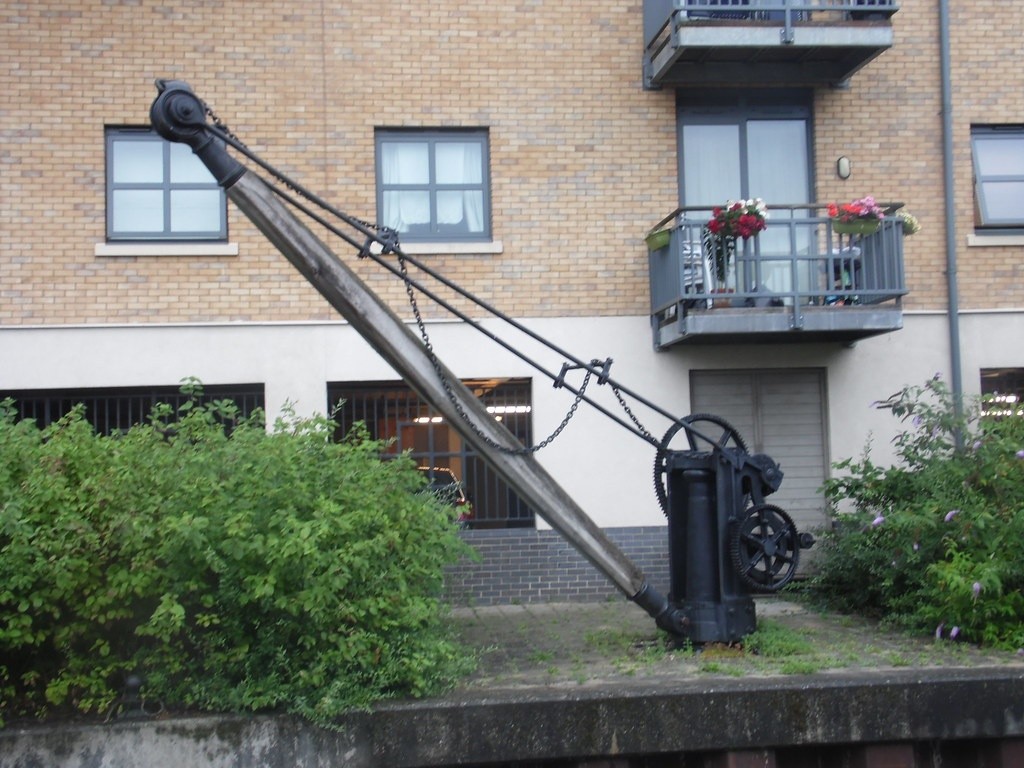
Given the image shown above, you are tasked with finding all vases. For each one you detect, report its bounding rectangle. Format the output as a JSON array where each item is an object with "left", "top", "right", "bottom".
[
  {"left": 830, "top": 217, "right": 880, "bottom": 234},
  {"left": 717, "top": 221, "right": 761, "bottom": 237},
  {"left": 644, "top": 228, "right": 671, "bottom": 252}
]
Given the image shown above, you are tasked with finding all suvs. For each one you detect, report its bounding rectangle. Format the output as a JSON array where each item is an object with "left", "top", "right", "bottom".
[{"left": 418, "top": 465, "right": 473, "bottom": 529}]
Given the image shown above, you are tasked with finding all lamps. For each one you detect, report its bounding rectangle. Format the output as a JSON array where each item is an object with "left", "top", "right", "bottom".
[{"left": 836, "top": 155, "right": 852, "bottom": 180}]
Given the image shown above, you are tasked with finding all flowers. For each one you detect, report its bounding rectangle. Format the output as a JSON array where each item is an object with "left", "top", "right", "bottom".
[
  {"left": 708, "top": 198, "right": 769, "bottom": 240},
  {"left": 827, "top": 195, "right": 886, "bottom": 223},
  {"left": 897, "top": 212, "right": 921, "bottom": 236}
]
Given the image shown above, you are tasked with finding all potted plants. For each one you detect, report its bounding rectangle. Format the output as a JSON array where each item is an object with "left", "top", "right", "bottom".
[
  {"left": 705, "top": 225, "right": 736, "bottom": 307},
  {"left": 825, "top": 244, "right": 864, "bottom": 305}
]
